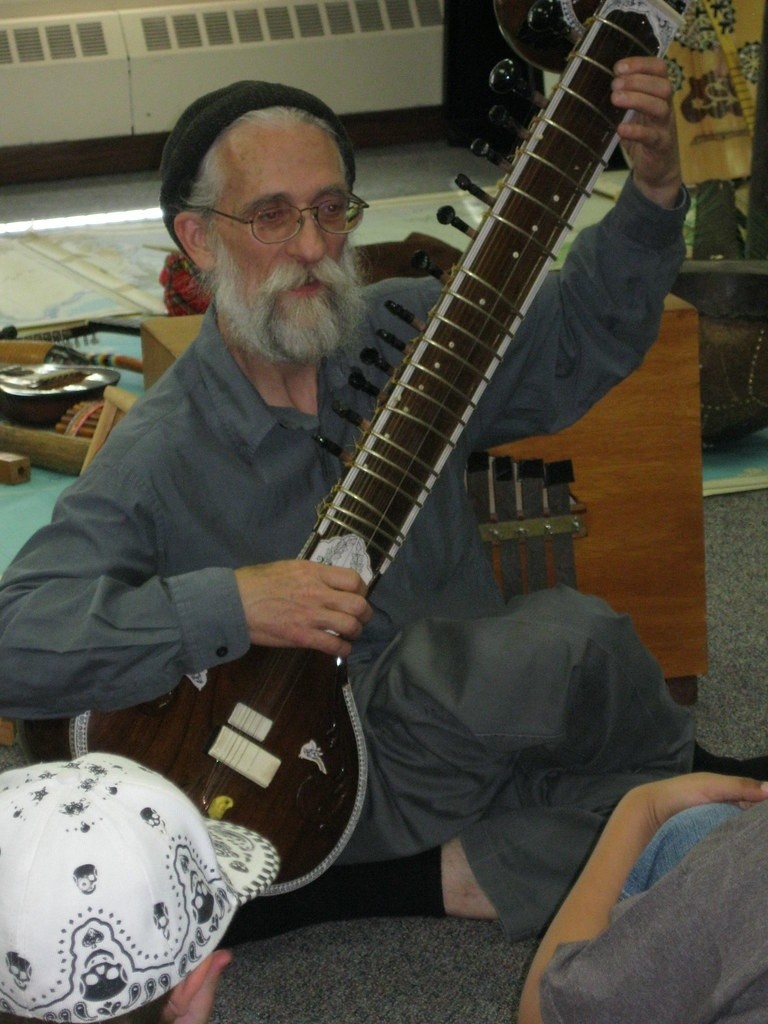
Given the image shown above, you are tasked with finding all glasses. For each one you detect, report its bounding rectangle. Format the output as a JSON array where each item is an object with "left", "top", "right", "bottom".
[{"left": 190, "top": 193, "right": 369, "bottom": 244}]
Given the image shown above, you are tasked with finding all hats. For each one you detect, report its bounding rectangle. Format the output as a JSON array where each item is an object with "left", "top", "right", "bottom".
[
  {"left": 0, "top": 753, "right": 281, "bottom": 1024},
  {"left": 161, "top": 80, "right": 356, "bottom": 246}
]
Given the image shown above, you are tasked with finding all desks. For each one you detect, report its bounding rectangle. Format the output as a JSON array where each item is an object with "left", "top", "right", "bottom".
[{"left": 139, "top": 299, "right": 711, "bottom": 707}]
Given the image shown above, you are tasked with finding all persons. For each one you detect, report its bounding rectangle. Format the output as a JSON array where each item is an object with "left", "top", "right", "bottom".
[
  {"left": 0, "top": 768, "right": 768, "bottom": 1024},
  {"left": 0, "top": 58, "right": 690, "bottom": 949}
]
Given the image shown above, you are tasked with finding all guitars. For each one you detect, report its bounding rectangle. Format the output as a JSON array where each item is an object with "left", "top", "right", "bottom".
[{"left": 12, "top": 0, "right": 697, "bottom": 897}]
